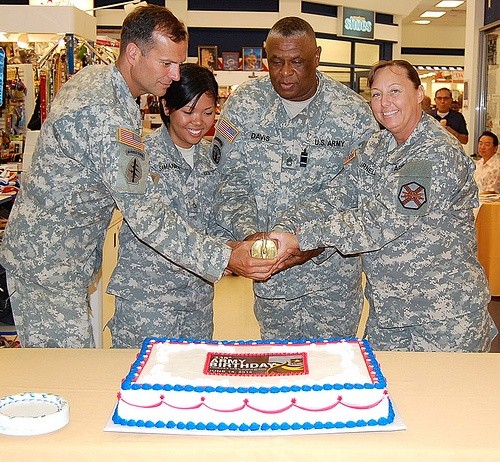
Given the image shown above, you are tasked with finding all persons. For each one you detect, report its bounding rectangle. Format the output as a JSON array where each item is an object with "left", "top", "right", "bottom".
[
  {"left": 270, "top": 60, "right": 499, "bottom": 353},
  {"left": 0, "top": 5, "right": 277, "bottom": 348},
  {"left": 210, "top": 16, "right": 380, "bottom": 340},
  {"left": 427, "top": 88, "right": 469, "bottom": 145},
  {"left": 474, "top": 131, "right": 500, "bottom": 194},
  {"left": 103, "top": 62, "right": 255, "bottom": 349}
]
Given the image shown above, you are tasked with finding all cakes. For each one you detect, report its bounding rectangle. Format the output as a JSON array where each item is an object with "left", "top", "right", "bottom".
[{"left": 112, "top": 337, "right": 395, "bottom": 431}]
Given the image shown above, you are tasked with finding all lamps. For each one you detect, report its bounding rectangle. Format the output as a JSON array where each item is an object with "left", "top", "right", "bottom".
[{"left": 17, "top": 33, "right": 28, "bottom": 48}]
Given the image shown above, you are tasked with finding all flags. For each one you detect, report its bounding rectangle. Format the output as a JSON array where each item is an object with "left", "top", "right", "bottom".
[
  {"left": 217, "top": 118, "right": 239, "bottom": 143},
  {"left": 343, "top": 148, "right": 355, "bottom": 164},
  {"left": 120, "top": 128, "right": 142, "bottom": 149}
]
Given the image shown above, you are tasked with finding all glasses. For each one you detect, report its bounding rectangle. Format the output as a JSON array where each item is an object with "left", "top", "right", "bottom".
[{"left": 436, "top": 97, "right": 451, "bottom": 101}]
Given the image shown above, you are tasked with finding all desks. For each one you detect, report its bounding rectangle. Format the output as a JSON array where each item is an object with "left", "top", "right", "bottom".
[{"left": 0, "top": 349, "right": 500, "bottom": 462}]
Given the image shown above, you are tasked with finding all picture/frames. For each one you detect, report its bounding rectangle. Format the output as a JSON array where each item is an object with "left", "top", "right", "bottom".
[
  {"left": 242, "top": 47, "right": 263, "bottom": 71},
  {"left": 222, "top": 52, "right": 241, "bottom": 70},
  {"left": 197, "top": 45, "right": 218, "bottom": 71}
]
